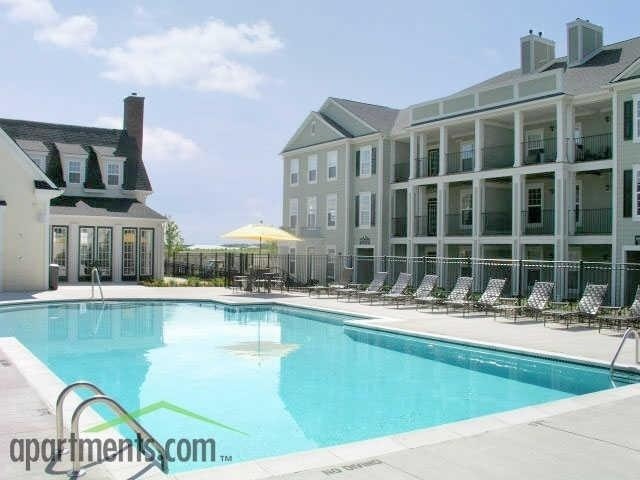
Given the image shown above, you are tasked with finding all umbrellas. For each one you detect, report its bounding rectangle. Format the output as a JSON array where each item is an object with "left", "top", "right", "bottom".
[{"left": 218, "top": 221, "right": 305, "bottom": 280}]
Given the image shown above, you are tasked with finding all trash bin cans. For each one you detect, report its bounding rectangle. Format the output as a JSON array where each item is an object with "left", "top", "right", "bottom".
[{"left": 49, "top": 264, "right": 59, "bottom": 290}]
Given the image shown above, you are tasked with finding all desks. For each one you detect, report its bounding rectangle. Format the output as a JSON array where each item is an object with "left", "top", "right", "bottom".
[{"left": 306, "top": 285, "right": 330, "bottom": 297}]
